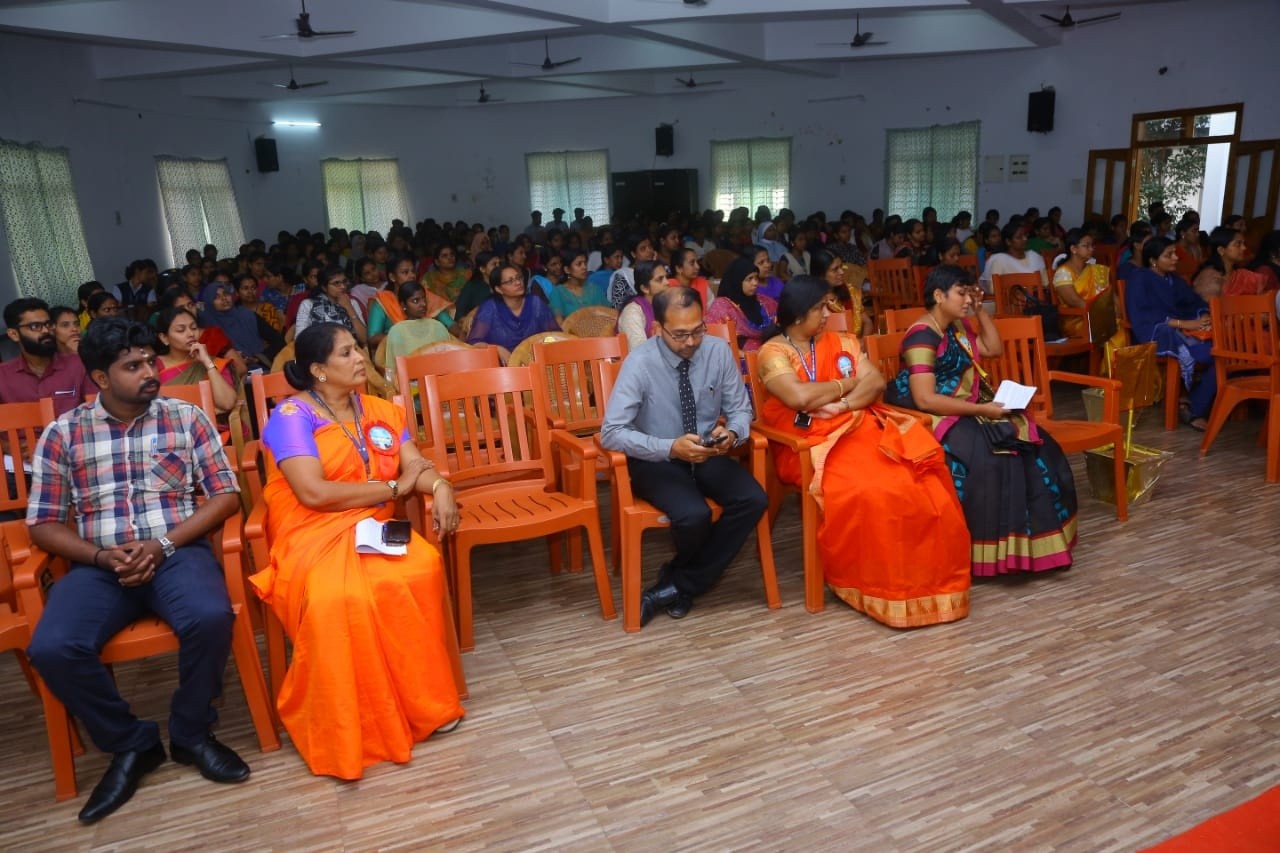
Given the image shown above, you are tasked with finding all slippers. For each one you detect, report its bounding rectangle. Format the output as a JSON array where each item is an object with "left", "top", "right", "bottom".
[{"left": 1179, "top": 414, "right": 1206, "bottom": 432}]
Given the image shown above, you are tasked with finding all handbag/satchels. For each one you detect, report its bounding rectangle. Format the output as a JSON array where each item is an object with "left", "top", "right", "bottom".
[
  {"left": 1010, "top": 283, "right": 1064, "bottom": 344},
  {"left": 1183, "top": 326, "right": 1214, "bottom": 340}
]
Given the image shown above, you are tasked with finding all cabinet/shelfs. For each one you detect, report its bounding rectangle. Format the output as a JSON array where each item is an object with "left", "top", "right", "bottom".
[{"left": 613, "top": 170, "right": 699, "bottom": 220}]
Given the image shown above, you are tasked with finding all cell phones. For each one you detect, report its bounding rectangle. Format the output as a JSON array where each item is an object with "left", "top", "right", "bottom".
[
  {"left": 704, "top": 435, "right": 729, "bottom": 446},
  {"left": 386, "top": 520, "right": 411, "bottom": 543},
  {"left": 794, "top": 414, "right": 812, "bottom": 426}
]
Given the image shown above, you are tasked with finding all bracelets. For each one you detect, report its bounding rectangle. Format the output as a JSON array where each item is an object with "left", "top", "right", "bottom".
[
  {"left": 432, "top": 479, "right": 450, "bottom": 493},
  {"left": 93, "top": 549, "right": 104, "bottom": 566},
  {"left": 840, "top": 398, "right": 849, "bottom": 410},
  {"left": 832, "top": 378, "right": 844, "bottom": 397}
]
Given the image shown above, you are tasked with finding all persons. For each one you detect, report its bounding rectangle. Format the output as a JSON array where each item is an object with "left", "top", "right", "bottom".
[
  {"left": 980, "top": 206, "right": 1150, "bottom": 377},
  {"left": 246, "top": 323, "right": 467, "bottom": 782},
  {"left": 0, "top": 240, "right": 288, "bottom": 521},
  {"left": 1137, "top": 202, "right": 1280, "bottom": 432},
  {"left": 627, "top": 203, "right": 755, "bottom": 277},
  {"left": 524, "top": 207, "right": 672, "bottom": 354},
  {"left": 665, "top": 246, "right": 785, "bottom": 409},
  {"left": 753, "top": 206, "right": 979, "bottom": 352},
  {"left": 896, "top": 264, "right": 1078, "bottom": 579},
  {"left": 597, "top": 286, "right": 768, "bottom": 629},
  {"left": 265, "top": 217, "right": 562, "bottom": 412},
  {"left": 758, "top": 274, "right": 972, "bottom": 625},
  {"left": 22, "top": 317, "right": 251, "bottom": 823}
]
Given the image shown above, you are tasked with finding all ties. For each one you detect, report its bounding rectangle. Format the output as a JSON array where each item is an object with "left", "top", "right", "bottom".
[{"left": 677, "top": 360, "right": 697, "bottom": 475}]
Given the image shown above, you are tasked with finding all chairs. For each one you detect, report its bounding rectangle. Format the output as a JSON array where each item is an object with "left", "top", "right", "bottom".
[{"left": 0, "top": 244, "right": 1280, "bottom": 802}]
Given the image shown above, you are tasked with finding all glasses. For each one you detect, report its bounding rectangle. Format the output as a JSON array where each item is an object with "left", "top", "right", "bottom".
[
  {"left": 661, "top": 319, "right": 707, "bottom": 342},
  {"left": 19, "top": 319, "right": 56, "bottom": 331},
  {"left": 328, "top": 278, "right": 349, "bottom": 288},
  {"left": 1075, "top": 243, "right": 1095, "bottom": 249},
  {"left": 501, "top": 273, "right": 524, "bottom": 285}
]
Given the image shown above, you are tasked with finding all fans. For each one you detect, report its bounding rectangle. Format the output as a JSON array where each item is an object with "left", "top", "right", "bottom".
[
  {"left": 816, "top": 12, "right": 891, "bottom": 48},
  {"left": 673, "top": 71, "right": 723, "bottom": 87},
  {"left": 457, "top": 83, "right": 506, "bottom": 103},
  {"left": 509, "top": 35, "right": 582, "bottom": 69},
  {"left": 272, "top": 63, "right": 327, "bottom": 90},
  {"left": 1039, "top": 4, "right": 1122, "bottom": 30},
  {"left": 261, "top": 0, "right": 357, "bottom": 38}
]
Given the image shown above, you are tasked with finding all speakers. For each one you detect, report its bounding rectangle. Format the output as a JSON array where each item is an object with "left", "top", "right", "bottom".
[
  {"left": 1028, "top": 91, "right": 1055, "bottom": 132},
  {"left": 256, "top": 139, "right": 279, "bottom": 172},
  {"left": 656, "top": 127, "right": 673, "bottom": 155}
]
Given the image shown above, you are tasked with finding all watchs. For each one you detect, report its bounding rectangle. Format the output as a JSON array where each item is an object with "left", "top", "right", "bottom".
[
  {"left": 386, "top": 480, "right": 399, "bottom": 501},
  {"left": 157, "top": 537, "right": 176, "bottom": 557}
]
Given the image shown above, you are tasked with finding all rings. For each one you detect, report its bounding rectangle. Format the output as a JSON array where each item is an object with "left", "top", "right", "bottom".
[{"left": 699, "top": 456, "right": 704, "bottom": 460}]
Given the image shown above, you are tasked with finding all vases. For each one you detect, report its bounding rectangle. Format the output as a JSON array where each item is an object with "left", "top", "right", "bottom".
[
  {"left": 1080, "top": 386, "right": 1145, "bottom": 430},
  {"left": 1083, "top": 441, "right": 1173, "bottom": 505}
]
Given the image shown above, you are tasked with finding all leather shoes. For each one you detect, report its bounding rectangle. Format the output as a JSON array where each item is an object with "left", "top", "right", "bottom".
[
  {"left": 666, "top": 598, "right": 692, "bottom": 618},
  {"left": 78, "top": 739, "right": 167, "bottom": 824},
  {"left": 169, "top": 734, "right": 250, "bottom": 784},
  {"left": 640, "top": 561, "right": 678, "bottom": 627}
]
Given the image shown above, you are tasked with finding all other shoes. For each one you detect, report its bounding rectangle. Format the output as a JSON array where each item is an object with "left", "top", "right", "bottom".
[{"left": 436, "top": 718, "right": 460, "bottom": 731}]
